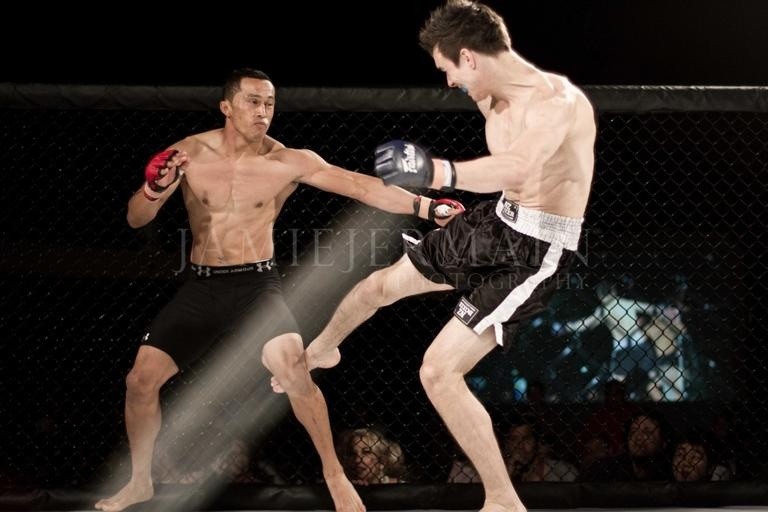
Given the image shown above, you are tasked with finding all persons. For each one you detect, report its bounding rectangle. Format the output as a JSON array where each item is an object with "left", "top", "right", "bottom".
[
  {"left": 552, "top": 281, "right": 679, "bottom": 402},
  {"left": 0, "top": 376, "right": 764, "bottom": 488},
  {"left": 638, "top": 299, "right": 694, "bottom": 400},
  {"left": 266, "top": 1, "right": 598, "bottom": 510},
  {"left": 92, "top": 67, "right": 465, "bottom": 510}
]
